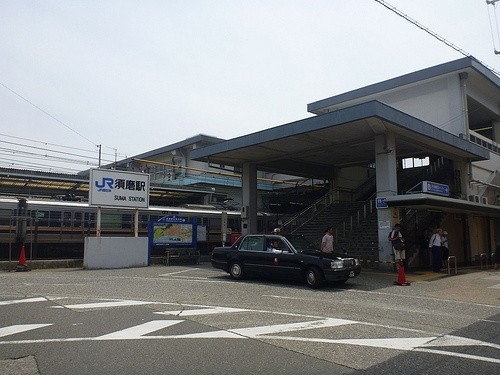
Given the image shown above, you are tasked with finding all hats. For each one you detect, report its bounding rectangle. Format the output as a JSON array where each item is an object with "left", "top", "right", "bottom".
[
  {"left": 435, "top": 228, "right": 442, "bottom": 234},
  {"left": 442, "top": 231, "right": 449, "bottom": 235},
  {"left": 394, "top": 224, "right": 401, "bottom": 227}
]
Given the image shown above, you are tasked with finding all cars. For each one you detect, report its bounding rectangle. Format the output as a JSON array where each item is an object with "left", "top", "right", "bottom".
[{"left": 210, "top": 233, "right": 361, "bottom": 289}]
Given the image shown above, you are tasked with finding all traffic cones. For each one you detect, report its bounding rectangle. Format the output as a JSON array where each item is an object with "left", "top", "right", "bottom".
[
  {"left": 394, "top": 259, "right": 411, "bottom": 286},
  {"left": 16, "top": 240, "right": 30, "bottom": 272}
]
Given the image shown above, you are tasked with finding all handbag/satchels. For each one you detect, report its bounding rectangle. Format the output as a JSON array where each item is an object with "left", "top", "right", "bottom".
[{"left": 393, "top": 237, "right": 406, "bottom": 250}]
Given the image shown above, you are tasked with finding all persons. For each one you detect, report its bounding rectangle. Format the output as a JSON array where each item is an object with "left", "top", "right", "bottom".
[
  {"left": 429, "top": 227, "right": 448, "bottom": 273},
  {"left": 271, "top": 240, "right": 282, "bottom": 255},
  {"left": 321, "top": 227, "right": 333, "bottom": 253},
  {"left": 388, "top": 223, "right": 406, "bottom": 273}
]
